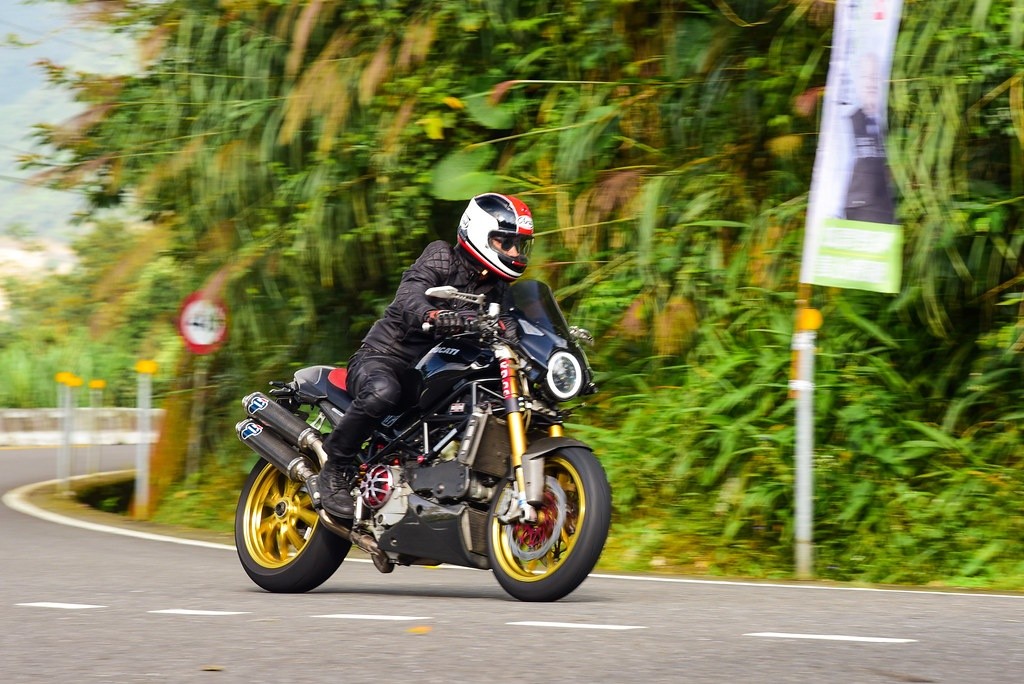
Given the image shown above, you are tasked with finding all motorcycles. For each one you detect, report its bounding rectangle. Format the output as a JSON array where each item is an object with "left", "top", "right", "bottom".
[{"left": 234, "top": 277, "right": 613, "bottom": 603}]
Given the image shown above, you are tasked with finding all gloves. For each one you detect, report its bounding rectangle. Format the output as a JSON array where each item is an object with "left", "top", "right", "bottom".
[{"left": 427, "top": 308, "right": 466, "bottom": 335}]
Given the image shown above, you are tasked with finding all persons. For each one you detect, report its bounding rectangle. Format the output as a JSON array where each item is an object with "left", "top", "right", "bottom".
[
  {"left": 315, "top": 191, "right": 534, "bottom": 520},
  {"left": 838, "top": 68, "right": 893, "bottom": 224}
]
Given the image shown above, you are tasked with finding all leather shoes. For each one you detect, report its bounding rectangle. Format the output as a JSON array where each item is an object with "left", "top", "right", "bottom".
[{"left": 316, "top": 459, "right": 355, "bottom": 520}]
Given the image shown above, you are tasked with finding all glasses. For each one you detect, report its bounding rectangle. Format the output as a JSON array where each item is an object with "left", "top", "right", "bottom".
[{"left": 492, "top": 232, "right": 530, "bottom": 254}]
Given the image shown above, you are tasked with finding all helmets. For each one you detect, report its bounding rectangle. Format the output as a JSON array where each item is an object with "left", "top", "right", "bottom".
[{"left": 457, "top": 192, "right": 534, "bottom": 282}]
